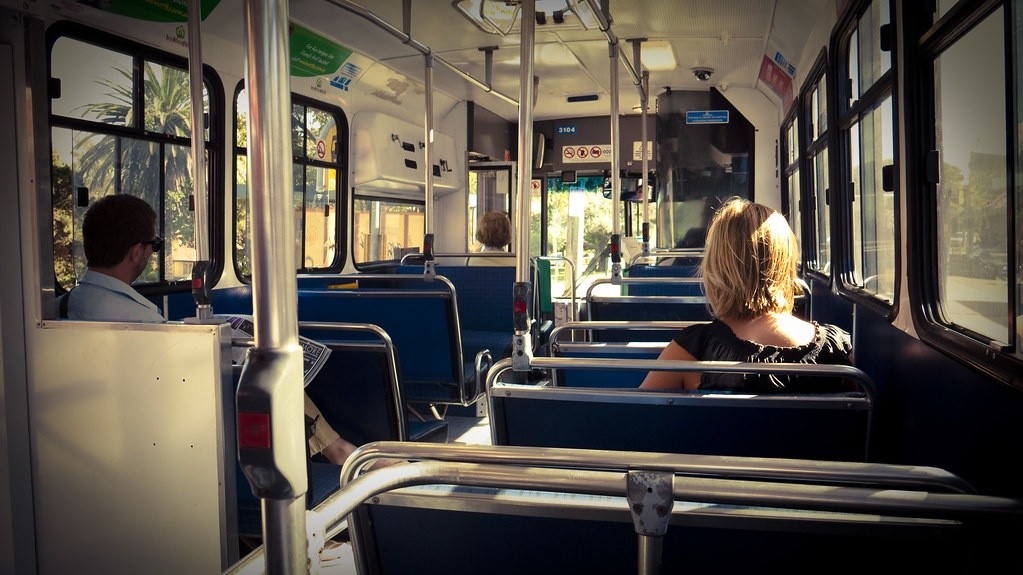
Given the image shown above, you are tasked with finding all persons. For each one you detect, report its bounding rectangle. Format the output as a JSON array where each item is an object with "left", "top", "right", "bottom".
[
  {"left": 55, "top": 194, "right": 406, "bottom": 472},
  {"left": 464, "top": 211, "right": 517, "bottom": 267},
  {"left": 639, "top": 196, "right": 854, "bottom": 395}
]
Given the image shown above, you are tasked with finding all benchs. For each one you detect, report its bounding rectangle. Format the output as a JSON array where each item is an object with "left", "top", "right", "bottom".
[{"left": 72, "top": 248, "right": 1023, "bottom": 575}]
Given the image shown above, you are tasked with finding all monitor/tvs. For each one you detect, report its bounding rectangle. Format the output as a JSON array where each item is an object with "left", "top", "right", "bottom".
[{"left": 561, "top": 170, "right": 577, "bottom": 185}]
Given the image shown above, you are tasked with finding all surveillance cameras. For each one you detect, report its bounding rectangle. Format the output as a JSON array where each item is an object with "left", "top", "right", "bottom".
[{"left": 691, "top": 67, "right": 713, "bottom": 81}]
